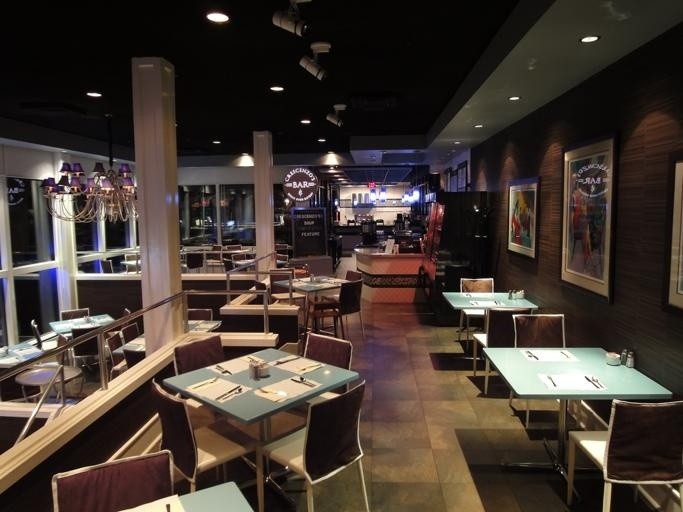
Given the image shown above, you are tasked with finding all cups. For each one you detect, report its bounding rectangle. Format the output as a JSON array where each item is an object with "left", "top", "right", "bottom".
[
  {"left": 255, "top": 363, "right": 269, "bottom": 378},
  {"left": 605, "top": 352, "right": 620, "bottom": 366}
]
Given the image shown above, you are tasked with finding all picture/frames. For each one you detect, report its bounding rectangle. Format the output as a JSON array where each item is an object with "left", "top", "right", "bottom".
[
  {"left": 558, "top": 135, "right": 614, "bottom": 304},
  {"left": 443, "top": 160, "right": 471, "bottom": 191},
  {"left": 506, "top": 176, "right": 538, "bottom": 263},
  {"left": 661, "top": 158, "right": 683, "bottom": 317}
]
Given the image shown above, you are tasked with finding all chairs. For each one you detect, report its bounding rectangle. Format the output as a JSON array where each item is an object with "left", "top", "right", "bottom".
[
  {"left": 511, "top": 313, "right": 581, "bottom": 430},
  {"left": 0, "top": 306, "right": 213, "bottom": 405},
  {"left": 472, "top": 307, "right": 533, "bottom": 395},
  {"left": 458, "top": 277, "right": 493, "bottom": 353},
  {"left": 301, "top": 330, "right": 353, "bottom": 393},
  {"left": 567, "top": 399, "right": 683, "bottom": 512},
  {"left": 253, "top": 269, "right": 365, "bottom": 343},
  {"left": 150, "top": 378, "right": 257, "bottom": 492},
  {"left": 173, "top": 336, "right": 224, "bottom": 417},
  {"left": 261, "top": 379, "right": 370, "bottom": 512},
  {"left": 51, "top": 448, "right": 174, "bottom": 511},
  {"left": 98, "top": 243, "right": 287, "bottom": 270}
]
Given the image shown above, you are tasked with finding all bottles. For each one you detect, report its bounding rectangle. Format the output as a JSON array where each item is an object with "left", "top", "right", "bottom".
[
  {"left": 248, "top": 358, "right": 255, "bottom": 380},
  {"left": 309, "top": 273, "right": 314, "bottom": 282},
  {"left": 619, "top": 348, "right": 628, "bottom": 364},
  {"left": 508, "top": 289, "right": 512, "bottom": 300},
  {"left": 253, "top": 363, "right": 262, "bottom": 381},
  {"left": 626, "top": 351, "right": 634, "bottom": 368},
  {"left": 511, "top": 290, "right": 516, "bottom": 299}
]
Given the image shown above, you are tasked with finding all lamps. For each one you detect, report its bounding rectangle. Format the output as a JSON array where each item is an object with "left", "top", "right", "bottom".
[
  {"left": 370, "top": 187, "right": 421, "bottom": 205},
  {"left": 325, "top": 105, "right": 347, "bottom": 127},
  {"left": 298, "top": 44, "right": 331, "bottom": 80},
  {"left": 273, "top": 0, "right": 317, "bottom": 39},
  {"left": 38, "top": 115, "right": 138, "bottom": 223}
]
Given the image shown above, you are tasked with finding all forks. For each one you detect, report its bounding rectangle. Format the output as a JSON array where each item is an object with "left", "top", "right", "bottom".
[
  {"left": 255, "top": 386, "right": 288, "bottom": 396},
  {"left": 192, "top": 375, "right": 219, "bottom": 389}
]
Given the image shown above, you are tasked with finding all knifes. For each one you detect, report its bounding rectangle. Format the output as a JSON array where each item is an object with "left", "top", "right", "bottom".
[
  {"left": 546, "top": 375, "right": 556, "bottom": 387},
  {"left": 289, "top": 377, "right": 312, "bottom": 389},
  {"left": 526, "top": 350, "right": 539, "bottom": 360},
  {"left": 213, "top": 385, "right": 241, "bottom": 400},
  {"left": 294, "top": 363, "right": 322, "bottom": 373},
  {"left": 560, "top": 351, "right": 569, "bottom": 358},
  {"left": 216, "top": 364, "right": 232, "bottom": 376},
  {"left": 583, "top": 375, "right": 598, "bottom": 390}
]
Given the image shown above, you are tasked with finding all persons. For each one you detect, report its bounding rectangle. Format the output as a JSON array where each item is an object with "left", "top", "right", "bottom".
[{"left": 511, "top": 200, "right": 533, "bottom": 245}]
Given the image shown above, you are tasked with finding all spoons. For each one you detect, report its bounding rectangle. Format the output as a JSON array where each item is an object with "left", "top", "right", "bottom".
[
  {"left": 272, "top": 356, "right": 302, "bottom": 366},
  {"left": 215, "top": 367, "right": 227, "bottom": 375},
  {"left": 222, "top": 387, "right": 243, "bottom": 401},
  {"left": 590, "top": 376, "right": 604, "bottom": 389},
  {"left": 299, "top": 375, "right": 316, "bottom": 387},
  {"left": 524, "top": 350, "right": 531, "bottom": 358}
]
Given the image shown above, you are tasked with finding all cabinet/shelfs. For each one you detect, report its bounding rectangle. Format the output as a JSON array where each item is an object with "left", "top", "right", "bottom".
[{"left": 416, "top": 192, "right": 499, "bottom": 323}]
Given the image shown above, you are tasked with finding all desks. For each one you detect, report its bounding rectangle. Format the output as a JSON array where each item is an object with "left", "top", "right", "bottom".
[
  {"left": 161, "top": 347, "right": 359, "bottom": 478},
  {"left": 120, "top": 479, "right": 252, "bottom": 511},
  {"left": 482, "top": 347, "right": 671, "bottom": 497},
  {"left": 442, "top": 292, "right": 538, "bottom": 360}
]
